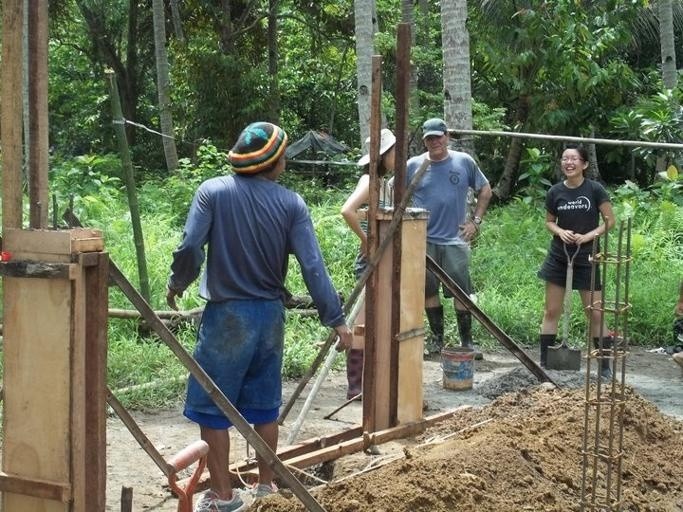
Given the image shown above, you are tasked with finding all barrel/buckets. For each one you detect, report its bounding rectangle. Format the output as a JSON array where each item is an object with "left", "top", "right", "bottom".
[{"left": 439, "top": 346, "right": 477, "bottom": 392}]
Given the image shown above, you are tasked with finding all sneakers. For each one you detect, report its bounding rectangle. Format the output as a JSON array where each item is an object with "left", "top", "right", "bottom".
[
  {"left": 193, "top": 490, "right": 249, "bottom": 512},
  {"left": 251, "top": 482, "right": 280, "bottom": 505}
]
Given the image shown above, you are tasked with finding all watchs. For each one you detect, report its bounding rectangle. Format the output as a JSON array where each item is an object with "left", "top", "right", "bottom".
[{"left": 471, "top": 216, "right": 483, "bottom": 226}]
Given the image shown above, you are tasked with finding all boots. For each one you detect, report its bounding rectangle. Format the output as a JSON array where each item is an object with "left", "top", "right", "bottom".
[
  {"left": 593, "top": 337, "right": 615, "bottom": 381},
  {"left": 424, "top": 305, "right": 445, "bottom": 355},
  {"left": 346, "top": 350, "right": 365, "bottom": 401},
  {"left": 454, "top": 309, "right": 484, "bottom": 360},
  {"left": 540, "top": 334, "right": 557, "bottom": 369}
]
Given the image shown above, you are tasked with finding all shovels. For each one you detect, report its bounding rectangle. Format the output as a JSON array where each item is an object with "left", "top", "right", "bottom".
[{"left": 546, "top": 242, "right": 581, "bottom": 370}]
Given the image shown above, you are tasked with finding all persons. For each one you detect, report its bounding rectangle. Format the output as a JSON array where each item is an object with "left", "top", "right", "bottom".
[
  {"left": 388, "top": 118, "right": 492, "bottom": 359},
  {"left": 539, "top": 145, "right": 615, "bottom": 375},
  {"left": 342, "top": 129, "right": 401, "bottom": 332},
  {"left": 167, "top": 122, "right": 352, "bottom": 512}
]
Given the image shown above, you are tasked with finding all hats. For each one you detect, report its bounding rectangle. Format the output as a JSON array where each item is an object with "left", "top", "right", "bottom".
[
  {"left": 228, "top": 122, "right": 287, "bottom": 176},
  {"left": 422, "top": 118, "right": 447, "bottom": 140},
  {"left": 357, "top": 128, "right": 397, "bottom": 166}
]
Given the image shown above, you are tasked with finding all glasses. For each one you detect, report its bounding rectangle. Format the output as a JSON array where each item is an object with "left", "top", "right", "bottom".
[{"left": 561, "top": 157, "right": 585, "bottom": 163}]
